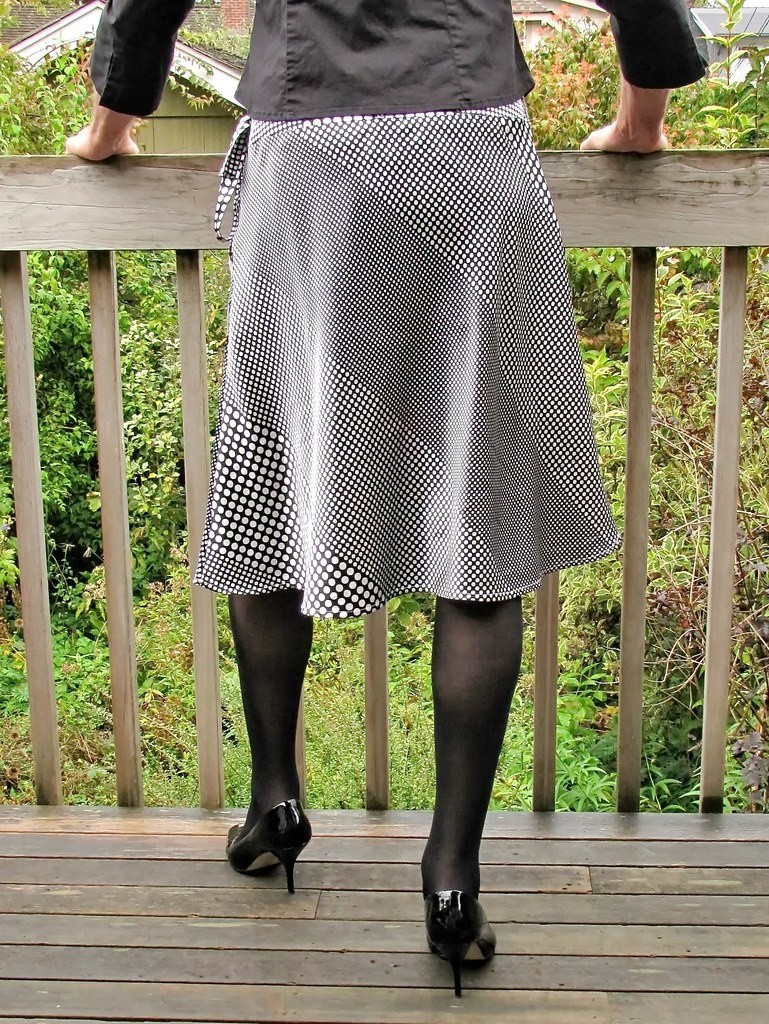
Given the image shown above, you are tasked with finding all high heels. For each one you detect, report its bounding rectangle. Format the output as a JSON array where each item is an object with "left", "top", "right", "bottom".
[
  {"left": 227, "top": 798, "right": 313, "bottom": 895},
  {"left": 425, "top": 890, "right": 495, "bottom": 997}
]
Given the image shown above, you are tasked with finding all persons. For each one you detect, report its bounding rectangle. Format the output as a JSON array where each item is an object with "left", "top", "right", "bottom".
[{"left": 66, "top": 0, "right": 715, "bottom": 1002}]
